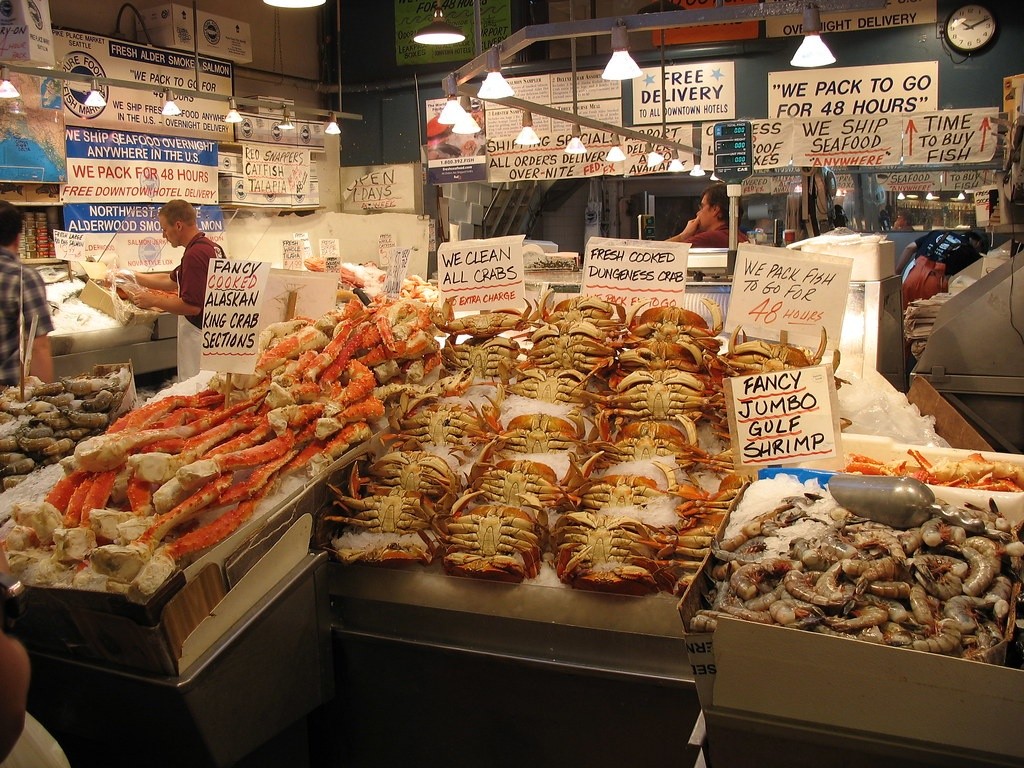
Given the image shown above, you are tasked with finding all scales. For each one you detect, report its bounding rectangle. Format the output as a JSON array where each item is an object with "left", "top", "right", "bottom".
[{"left": 688, "top": 120, "right": 754, "bottom": 273}]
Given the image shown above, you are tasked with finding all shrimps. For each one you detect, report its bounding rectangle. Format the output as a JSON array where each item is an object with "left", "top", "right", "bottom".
[
  {"left": 687, "top": 491, "right": 1024, "bottom": 659},
  {"left": 1, "top": 362, "right": 130, "bottom": 486}
]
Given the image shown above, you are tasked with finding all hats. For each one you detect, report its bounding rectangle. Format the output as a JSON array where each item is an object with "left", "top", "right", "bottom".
[{"left": 972, "top": 229, "right": 990, "bottom": 254}]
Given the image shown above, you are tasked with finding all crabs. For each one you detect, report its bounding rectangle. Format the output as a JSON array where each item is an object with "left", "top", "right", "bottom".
[
  {"left": 5, "top": 259, "right": 446, "bottom": 590},
  {"left": 841, "top": 447, "right": 1024, "bottom": 496},
  {"left": 322, "top": 290, "right": 855, "bottom": 599}
]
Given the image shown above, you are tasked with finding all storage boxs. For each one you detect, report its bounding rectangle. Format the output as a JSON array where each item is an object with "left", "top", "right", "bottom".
[
  {"left": 805, "top": 240, "right": 895, "bottom": 281},
  {"left": 677, "top": 470, "right": 1024, "bottom": 768}
]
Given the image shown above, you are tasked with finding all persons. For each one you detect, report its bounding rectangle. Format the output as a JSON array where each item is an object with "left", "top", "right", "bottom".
[
  {"left": 0, "top": 199, "right": 55, "bottom": 387},
  {"left": 665, "top": 184, "right": 750, "bottom": 248},
  {"left": 896, "top": 230, "right": 989, "bottom": 365},
  {"left": 879, "top": 209, "right": 932, "bottom": 231},
  {"left": 112, "top": 199, "right": 227, "bottom": 382}
]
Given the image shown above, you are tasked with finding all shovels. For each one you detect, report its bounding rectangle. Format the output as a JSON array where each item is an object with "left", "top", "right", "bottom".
[{"left": 829, "top": 475, "right": 992, "bottom": 536}]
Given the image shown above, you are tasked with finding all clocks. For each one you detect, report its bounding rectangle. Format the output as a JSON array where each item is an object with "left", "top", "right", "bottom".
[{"left": 944, "top": 3, "right": 997, "bottom": 57}]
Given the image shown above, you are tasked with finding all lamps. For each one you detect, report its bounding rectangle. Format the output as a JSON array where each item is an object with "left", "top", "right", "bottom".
[{"left": 0, "top": 0, "right": 966, "bottom": 202}]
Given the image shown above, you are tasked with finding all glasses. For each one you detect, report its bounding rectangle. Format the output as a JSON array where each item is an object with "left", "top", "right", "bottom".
[{"left": 698, "top": 203, "right": 710, "bottom": 212}]
